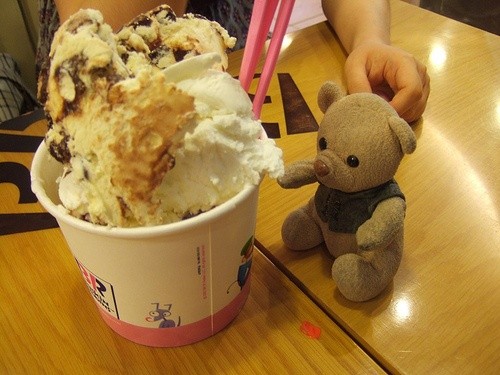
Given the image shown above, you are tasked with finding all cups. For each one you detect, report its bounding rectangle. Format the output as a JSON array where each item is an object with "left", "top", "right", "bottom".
[{"left": 29, "top": 139, "right": 264, "bottom": 346}]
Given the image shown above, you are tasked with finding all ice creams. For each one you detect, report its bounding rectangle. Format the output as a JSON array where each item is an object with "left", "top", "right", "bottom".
[{"left": 36, "top": 3, "right": 284, "bottom": 229}]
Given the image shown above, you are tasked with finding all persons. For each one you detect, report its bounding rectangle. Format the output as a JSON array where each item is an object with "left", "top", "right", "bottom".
[{"left": 35, "top": 0, "right": 432, "bottom": 130}]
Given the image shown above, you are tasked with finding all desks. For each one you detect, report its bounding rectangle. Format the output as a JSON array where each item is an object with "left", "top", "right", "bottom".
[{"left": 1, "top": 0, "right": 499, "bottom": 375}]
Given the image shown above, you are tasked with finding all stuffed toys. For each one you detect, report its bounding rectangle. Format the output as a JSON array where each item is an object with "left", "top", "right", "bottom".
[{"left": 276, "top": 80, "right": 419, "bottom": 304}]
